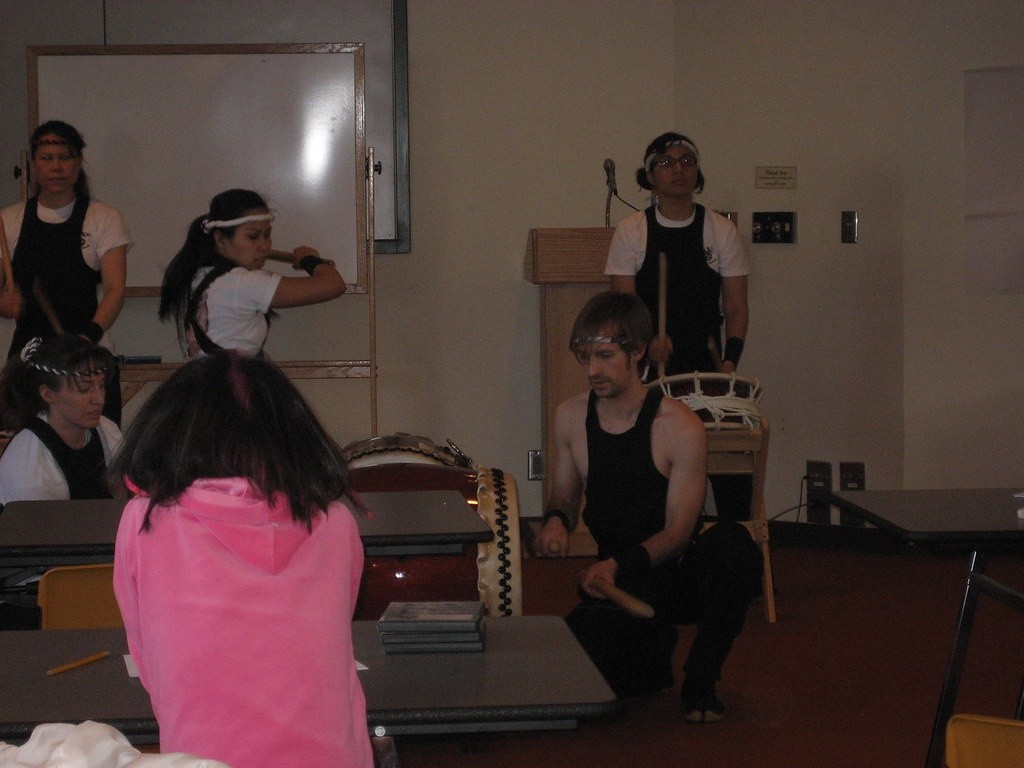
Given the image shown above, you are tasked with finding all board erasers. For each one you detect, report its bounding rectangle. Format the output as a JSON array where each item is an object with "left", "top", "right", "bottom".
[{"left": 123, "top": 351, "right": 160, "bottom": 365}]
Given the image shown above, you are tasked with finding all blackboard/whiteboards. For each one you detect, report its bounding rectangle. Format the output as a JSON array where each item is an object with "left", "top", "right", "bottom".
[
  {"left": 26, "top": 42, "right": 368, "bottom": 293},
  {"left": 1, "top": 0, "right": 412, "bottom": 254}
]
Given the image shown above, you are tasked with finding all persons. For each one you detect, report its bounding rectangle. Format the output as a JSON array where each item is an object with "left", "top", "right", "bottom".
[
  {"left": 531, "top": 290, "right": 765, "bottom": 721},
  {"left": 113, "top": 353, "right": 377, "bottom": 767},
  {"left": 0, "top": 333, "right": 130, "bottom": 630},
  {"left": 157, "top": 189, "right": 347, "bottom": 356},
  {"left": 604, "top": 131, "right": 757, "bottom": 521},
  {"left": 0, "top": 118, "right": 134, "bottom": 433}
]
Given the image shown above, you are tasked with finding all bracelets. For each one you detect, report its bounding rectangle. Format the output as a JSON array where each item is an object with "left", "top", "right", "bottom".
[
  {"left": 723, "top": 336, "right": 743, "bottom": 364},
  {"left": 299, "top": 255, "right": 329, "bottom": 275},
  {"left": 78, "top": 321, "right": 103, "bottom": 343},
  {"left": 543, "top": 509, "right": 569, "bottom": 529}
]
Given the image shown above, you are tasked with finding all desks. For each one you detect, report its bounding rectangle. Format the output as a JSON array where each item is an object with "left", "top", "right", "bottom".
[
  {"left": 824, "top": 489, "right": 1024, "bottom": 768},
  {"left": 0, "top": 488, "right": 618, "bottom": 768}
]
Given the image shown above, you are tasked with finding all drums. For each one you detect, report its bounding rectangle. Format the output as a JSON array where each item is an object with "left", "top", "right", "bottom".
[
  {"left": 673, "top": 382, "right": 748, "bottom": 423},
  {"left": 342, "top": 461, "right": 524, "bottom": 622},
  {"left": 341, "top": 430, "right": 474, "bottom": 470}
]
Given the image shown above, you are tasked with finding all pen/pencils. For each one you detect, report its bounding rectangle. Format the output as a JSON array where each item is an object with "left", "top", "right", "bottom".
[{"left": 46, "top": 648, "right": 109, "bottom": 674}]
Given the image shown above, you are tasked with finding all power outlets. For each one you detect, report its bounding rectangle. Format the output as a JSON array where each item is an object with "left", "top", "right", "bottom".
[
  {"left": 806, "top": 460, "right": 833, "bottom": 526},
  {"left": 840, "top": 461, "right": 866, "bottom": 528},
  {"left": 528, "top": 450, "right": 544, "bottom": 480},
  {"left": 841, "top": 210, "right": 858, "bottom": 243}
]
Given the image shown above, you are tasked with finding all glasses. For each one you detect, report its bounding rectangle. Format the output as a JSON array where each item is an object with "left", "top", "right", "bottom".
[{"left": 652, "top": 154, "right": 698, "bottom": 169}]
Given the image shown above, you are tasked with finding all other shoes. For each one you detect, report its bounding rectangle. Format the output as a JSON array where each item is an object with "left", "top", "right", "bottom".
[{"left": 681, "top": 676, "right": 723, "bottom": 723}]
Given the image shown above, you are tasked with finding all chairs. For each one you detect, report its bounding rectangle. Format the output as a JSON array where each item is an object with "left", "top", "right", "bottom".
[
  {"left": 642, "top": 415, "right": 781, "bottom": 625},
  {"left": 38, "top": 562, "right": 128, "bottom": 631}
]
